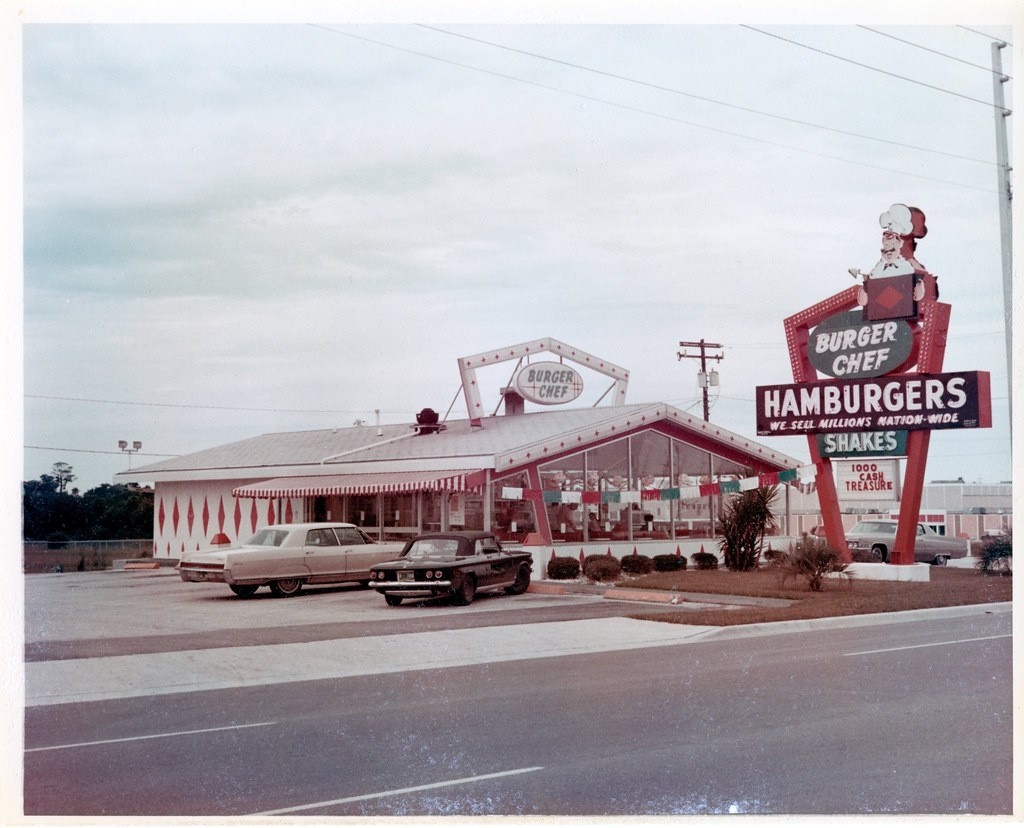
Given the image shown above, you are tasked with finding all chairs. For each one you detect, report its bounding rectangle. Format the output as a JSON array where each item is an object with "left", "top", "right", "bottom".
[{"left": 879, "top": 524, "right": 892, "bottom": 533}]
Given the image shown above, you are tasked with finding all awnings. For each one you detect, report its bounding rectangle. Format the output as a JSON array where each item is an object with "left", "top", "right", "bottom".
[{"left": 232, "top": 469, "right": 483, "bottom": 498}]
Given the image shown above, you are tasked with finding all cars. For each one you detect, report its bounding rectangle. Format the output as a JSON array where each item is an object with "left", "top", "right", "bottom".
[
  {"left": 813, "top": 519, "right": 969, "bottom": 566},
  {"left": 368, "top": 531, "right": 532, "bottom": 606},
  {"left": 174, "top": 520, "right": 419, "bottom": 599}
]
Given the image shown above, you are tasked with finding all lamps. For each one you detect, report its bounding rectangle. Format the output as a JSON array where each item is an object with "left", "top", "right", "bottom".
[
  {"left": 688, "top": 520, "right": 694, "bottom": 538},
  {"left": 560, "top": 523, "right": 567, "bottom": 539},
  {"left": 648, "top": 522, "right": 653, "bottom": 537},
  {"left": 512, "top": 522, "right": 517, "bottom": 541},
  {"left": 605, "top": 522, "right": 611, "bottom": 538}
]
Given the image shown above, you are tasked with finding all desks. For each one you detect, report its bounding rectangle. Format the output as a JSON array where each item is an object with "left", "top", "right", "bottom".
[{"left": 498, "top": 530, "right": 706, "bottom": 543}]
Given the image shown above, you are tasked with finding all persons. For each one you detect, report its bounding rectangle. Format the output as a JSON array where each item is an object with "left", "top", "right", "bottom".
[
  {"left": 587, "top": 513, "right": 602, "bottom": 537},
  {"left": 557, "top": 502, "right": 578, "bottom": 533}
]
